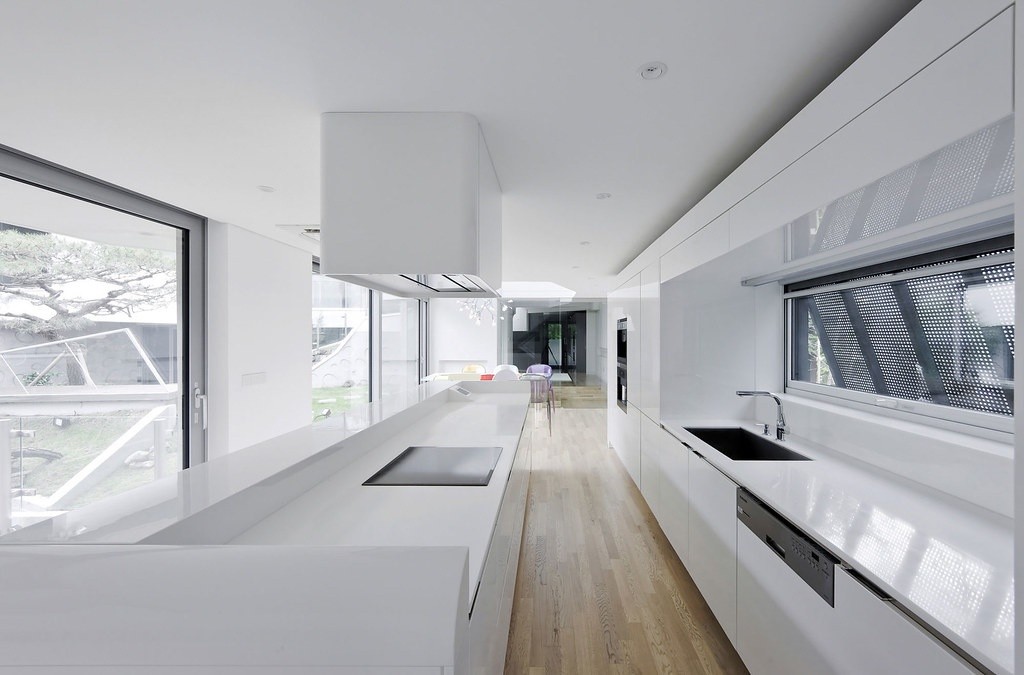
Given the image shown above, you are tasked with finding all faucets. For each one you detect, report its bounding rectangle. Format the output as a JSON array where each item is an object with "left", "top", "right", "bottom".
[{"left": 736, "top": 390, "right": 788, "bottom": 442}]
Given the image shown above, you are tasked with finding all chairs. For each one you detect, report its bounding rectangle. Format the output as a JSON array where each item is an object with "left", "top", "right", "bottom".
[
  {"left": 461, "top": 363, "right": 486, "bottom": 374},
  {"left": 527, "top": 364, "right": 556, "bottom": 414},
  {"left": 519, "top": 374, "right": 551, "bottom": 436},
  {"left": 492, "top": 369, "right": 518, "bottom": 381},
  {"left": 493, "top": 365, "right": 518, "bottom": 374}
]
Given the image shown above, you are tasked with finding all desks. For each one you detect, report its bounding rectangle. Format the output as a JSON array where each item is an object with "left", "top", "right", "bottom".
[{"left": 421, "top": 373, "right": 572, "bottom": 428}]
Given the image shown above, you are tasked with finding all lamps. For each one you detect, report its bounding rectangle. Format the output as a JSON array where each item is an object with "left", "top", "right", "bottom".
[{"left": 456, "top": 298, "right": 515, "bottom": 327}]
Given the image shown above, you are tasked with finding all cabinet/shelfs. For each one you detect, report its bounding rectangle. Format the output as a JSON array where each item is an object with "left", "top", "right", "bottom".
[{"left": 657, "top": 427, "right": 740, "bottom": 649}]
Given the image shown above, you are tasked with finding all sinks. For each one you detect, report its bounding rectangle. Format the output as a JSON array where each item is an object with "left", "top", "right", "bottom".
[{"left": 682, "top": 427, "right": 816, "bottom": 461}]
